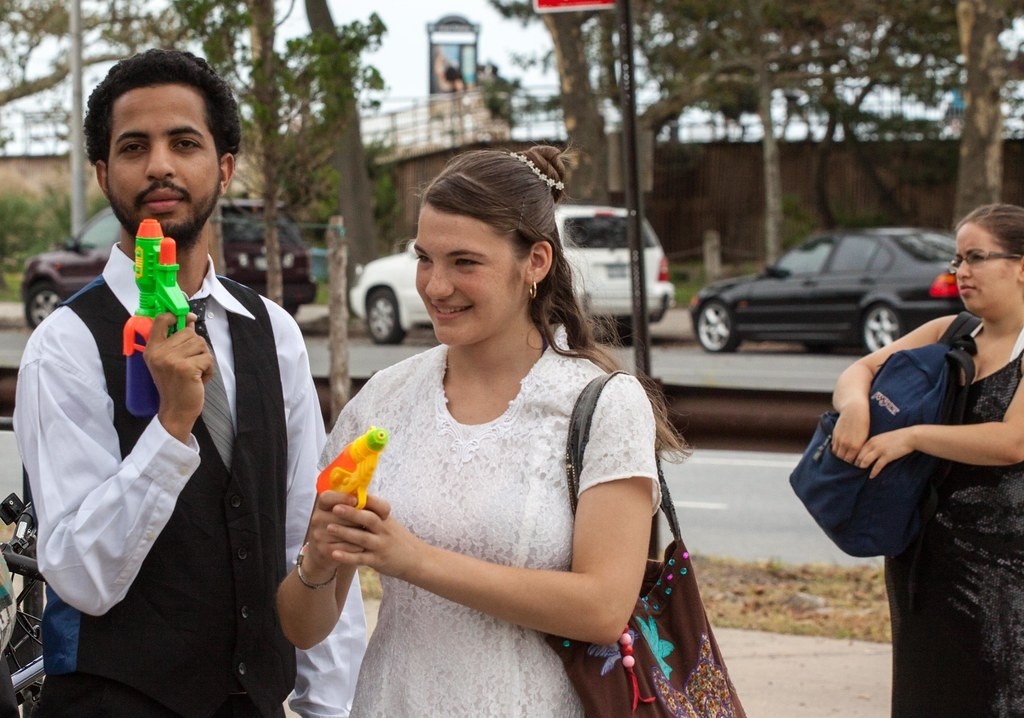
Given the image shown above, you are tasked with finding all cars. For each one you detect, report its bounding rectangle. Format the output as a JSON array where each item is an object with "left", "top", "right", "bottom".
[{"left": 688, "top": 229, "right": 969, "bottom": 359}]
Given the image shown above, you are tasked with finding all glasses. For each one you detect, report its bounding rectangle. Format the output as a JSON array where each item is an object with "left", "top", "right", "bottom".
[{"left": 948, "top": 250, "right": 1021, "bottom": 273}]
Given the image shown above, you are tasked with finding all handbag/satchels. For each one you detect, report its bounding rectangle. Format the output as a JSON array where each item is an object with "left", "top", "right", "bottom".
[{"left": 545, "top": 369, "right": 749, "bottom": 718}]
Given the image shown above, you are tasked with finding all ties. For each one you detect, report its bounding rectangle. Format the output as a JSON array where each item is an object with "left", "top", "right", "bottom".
[{"left": 173, "top": 295, "right": 236, "bottom": 478}]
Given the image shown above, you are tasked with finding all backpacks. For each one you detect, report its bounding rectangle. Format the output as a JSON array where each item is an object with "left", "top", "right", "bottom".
[{"left": 789, "top": 311, "right": 983, "bottom": 558}]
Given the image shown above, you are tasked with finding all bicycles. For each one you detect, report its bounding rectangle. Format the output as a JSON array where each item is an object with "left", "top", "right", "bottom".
[{"left": 0, "top": 494, "right": 58, "bottom": 717}]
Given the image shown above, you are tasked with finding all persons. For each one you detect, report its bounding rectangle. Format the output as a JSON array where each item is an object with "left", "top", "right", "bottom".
[
  {"left": 275, "top": 146, "right": 692, "bottom": 718},
  {"left": 12, "top": 50, "right": 366, "bottom": 718},
  {"left": 832, "top": 203, "right": 1024, "bottom": 718}
]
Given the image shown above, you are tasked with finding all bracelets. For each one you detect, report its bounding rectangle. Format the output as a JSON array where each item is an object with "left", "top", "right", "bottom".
[{"left": 296, "top": 542, "right": 338, "bottom": 589}]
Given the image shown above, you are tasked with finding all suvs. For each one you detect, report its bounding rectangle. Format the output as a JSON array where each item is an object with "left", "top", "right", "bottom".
[
  {"left": 19, "top": 198, "right": 318, "bottom": 332},
  {"left": 345, "top": 206, "right": 676, "bottom": 342}
]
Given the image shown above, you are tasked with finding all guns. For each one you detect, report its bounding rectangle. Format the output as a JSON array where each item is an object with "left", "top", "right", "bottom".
[
  {"left": 315, "top": 422, "right": 387, "bottom": 510},
  {"left": 119, "top": 217, "right": 190, "bottom": 418}
]
